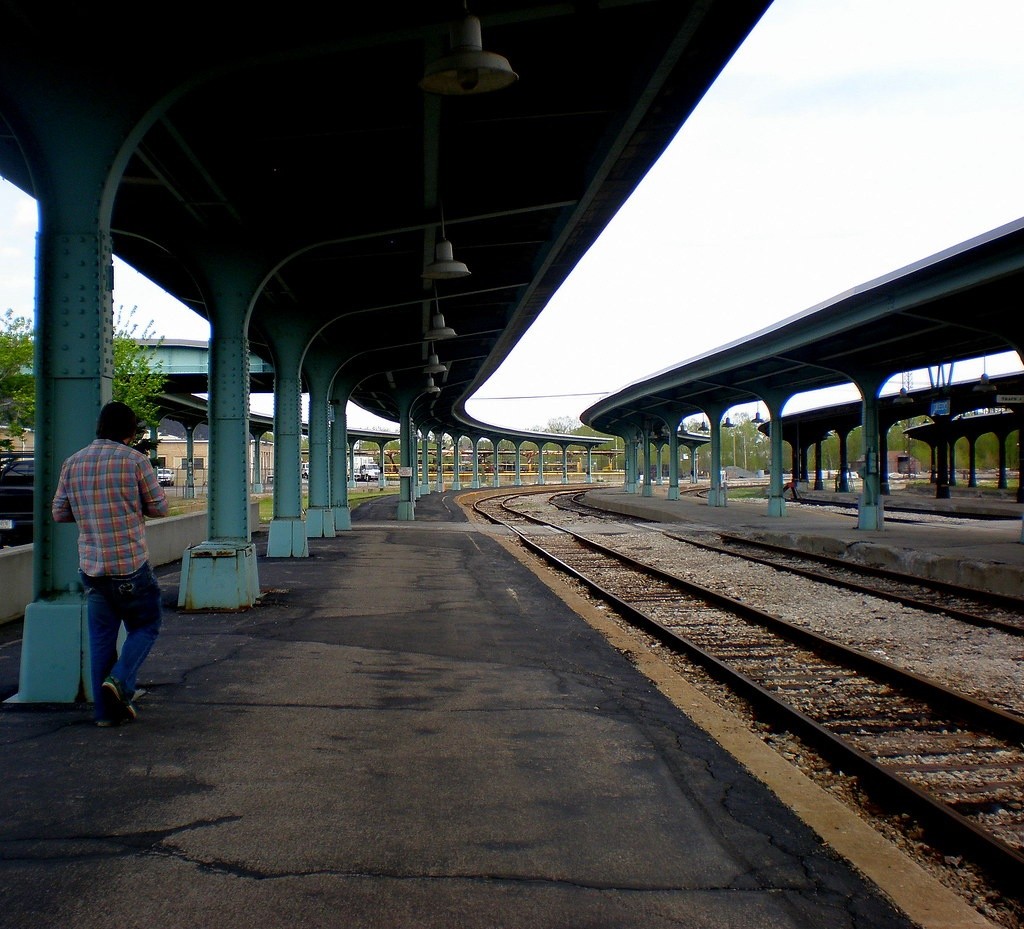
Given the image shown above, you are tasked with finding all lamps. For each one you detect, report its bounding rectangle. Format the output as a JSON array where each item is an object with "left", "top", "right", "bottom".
[
  {"left": 722, "top": 408, "right": 735, "bottom": 428},
  {"left": 751, "top": 398, "right": 765, "bottom": 423},
  {"left": 420, "top": 199, "right": 471, "bottom": 280},
  {"left": 423, "top": 340, "right": 449, "bottom": 374},
  {"left": 422, "top": 0, "right": 522, "bottom": 95},
  {"left": 698, "top": 411, "right": 710, "bottom": 431},
  {"left": 425, "top": 373, "right": 441, "bottom": 394},
  {"left": 893, "top": 358, "right": 914, "bottom": 405},
  {"left": 423, "top": 280, "right": 458, "bottom": 341},
  {"left": 973, "top": 320, "right": 997, "bottom": 393},
  {"left": 677, "top": 411, "right": 689, "bottom": 434}
]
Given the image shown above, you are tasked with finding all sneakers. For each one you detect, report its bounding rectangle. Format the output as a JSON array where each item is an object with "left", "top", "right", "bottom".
[
  {"left": 101, "top": 675, "right": 137, "bottom": 719},
  {"left": 94, "top": 717, "right": 113, "bottom": 726}
]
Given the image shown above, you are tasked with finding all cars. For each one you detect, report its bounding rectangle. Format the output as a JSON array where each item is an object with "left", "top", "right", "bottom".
[
  {"left": 157, "top": 468, "right": 175, "bottom": 487},
  {"left": 0, "top": 459, "right": 34, "bottom": 547},
  {"left": 302, "top": 462, "right": 309, "bottom": 479}
]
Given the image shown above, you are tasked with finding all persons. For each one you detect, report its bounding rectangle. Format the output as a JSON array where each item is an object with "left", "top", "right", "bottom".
[{"left": 52, "top": 401, "right": 168, "bottom": 728}]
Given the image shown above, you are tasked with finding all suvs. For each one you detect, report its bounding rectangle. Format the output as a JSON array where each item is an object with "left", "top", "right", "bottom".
[{"left": 360, "top": 464, "right": 380, "bottom": 482}]
